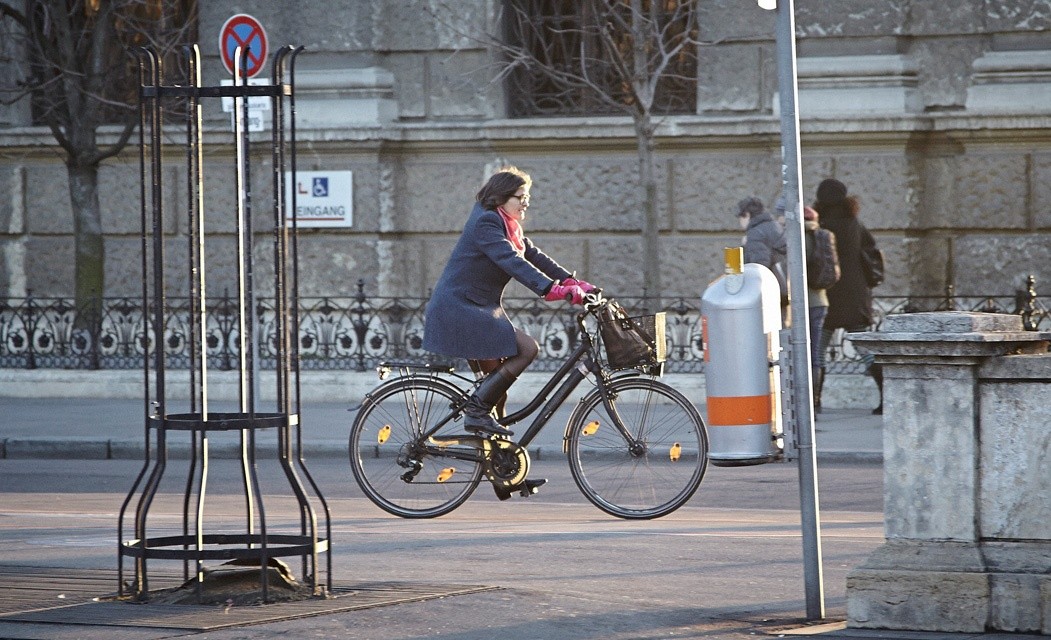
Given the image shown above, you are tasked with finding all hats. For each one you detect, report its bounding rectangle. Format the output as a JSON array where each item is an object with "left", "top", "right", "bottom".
[
  {"left": 734, "top": 196, "right": 764, "bottom": 217},
  {"left": 817, "top": 179, "right": 846, "bottom": 200},
  {"left": 777, "top": 195, "right": 784, "bottom": 210}
]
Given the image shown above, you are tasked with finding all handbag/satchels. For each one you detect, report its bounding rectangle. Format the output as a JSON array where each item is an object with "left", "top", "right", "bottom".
[
  {"left": 584, "top": 292, "right": 655, "bottom": 369},
  {"left": 860, "top": 224, "right": 884, "bottom": 287}
]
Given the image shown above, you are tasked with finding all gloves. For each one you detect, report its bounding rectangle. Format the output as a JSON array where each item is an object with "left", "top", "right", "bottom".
[
  {"left": 545, "top": 279, "right": 585, "bottom": 305},
  {"left": 561, "top": 271, "right": 600, "bottom": 294}
]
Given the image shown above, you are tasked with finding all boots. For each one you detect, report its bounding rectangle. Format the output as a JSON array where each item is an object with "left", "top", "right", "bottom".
[
  {"left": 813, "top": 367, "right": 825, "bottom": 409},
  {"left": 866, "top": 361, "right": 883, "bottom": 414},
  {"left": 464, "top": 368, "right": 514, "bottom": 434},
  {"left": 492, "top": 479, "right": 548, "bottom": 501}
]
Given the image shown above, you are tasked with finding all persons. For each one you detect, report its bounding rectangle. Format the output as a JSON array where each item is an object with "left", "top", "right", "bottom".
[
  {"left": 810, "top": 178, "right": 886, "bottom": 416},
  {"left": 421, "top": 167, "right": 598, "bottom": 438},
  {"left": 735, "top": 195, "right": 789, "bottom": 331},
  {"left": 776, "top": 195, "right": 841, "bottom": 422}
]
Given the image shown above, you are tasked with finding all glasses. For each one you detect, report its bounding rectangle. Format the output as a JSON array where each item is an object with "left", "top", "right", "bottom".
[{"left": 510, "top": 193, "right": 531, "bottom": 206}]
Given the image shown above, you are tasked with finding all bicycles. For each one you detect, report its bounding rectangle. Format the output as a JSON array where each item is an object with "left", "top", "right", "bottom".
[{"left": 346, "top": 286, "right": 708, "bottom": 519}]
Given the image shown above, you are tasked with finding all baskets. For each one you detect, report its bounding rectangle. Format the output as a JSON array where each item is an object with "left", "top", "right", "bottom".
[{"left": 596, "top": 313, "right": 660, "bottom": 370}]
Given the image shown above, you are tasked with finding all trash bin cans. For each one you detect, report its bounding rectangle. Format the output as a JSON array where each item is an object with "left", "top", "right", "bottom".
[{"left": 701, "top": 262, "right": 785, "bottom": 467}]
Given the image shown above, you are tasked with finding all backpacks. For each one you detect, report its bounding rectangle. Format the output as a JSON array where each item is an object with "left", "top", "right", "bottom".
[{"left": 807, "top": 228, "right": 840, "bottom": 289}]
